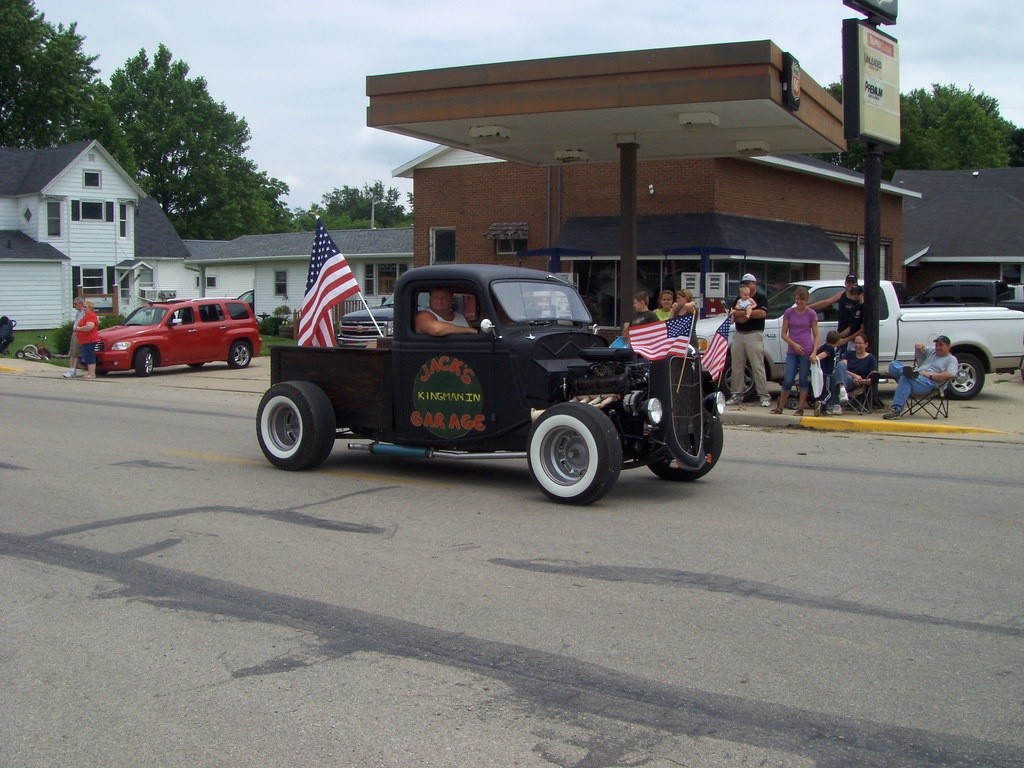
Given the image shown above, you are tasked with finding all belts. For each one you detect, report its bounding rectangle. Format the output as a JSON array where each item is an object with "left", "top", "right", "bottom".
[{"left": 737, "top": 329, "right": 764, "bottom": 334}]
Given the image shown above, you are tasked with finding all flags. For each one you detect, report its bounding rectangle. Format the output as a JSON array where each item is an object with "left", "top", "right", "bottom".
[
  {"left": 699, "top": 315, "right": 731, "bottom": 382},
  {"left": 627, "top": 316, "right": 694, "bottom": 361},
  {"left": 298, "top": 221, "right": 360, "bottom": 349}
]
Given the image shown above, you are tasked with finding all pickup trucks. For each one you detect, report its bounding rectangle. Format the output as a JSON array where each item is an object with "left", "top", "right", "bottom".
[
  {"left": 906, "top": 279, "right": 1024, "bottom": 314},
  {"left": 255, "top": 262, "right": 727, "bottom": 506},
  {"left": 691, "top": 279, "right": 1024, "bottom": 403}
]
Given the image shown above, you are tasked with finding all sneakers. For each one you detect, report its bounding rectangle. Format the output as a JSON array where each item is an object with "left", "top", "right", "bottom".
[
  {"left": 838, "top": 391, "right": 849, "bottom": 403},
  {"left": 726, "top": 394, "right": 742, "bottom": 405},
  {"left": 902, "top": 366, "right": 919, "bottom": 378},
  {"left": 883, "top": 409, "right": 901, "bottom": 420},
  {"left": 762, "top": 400, "right": 771, "bottom": 407},
  {"left": 820, "top": 410, "right": 832, "bottom": 416},
  {"left": 815, "top": 401, "right": 822, "bottom": 416},
  {"left": 833, "top": 404, "right": 842, "bottom": 415}
]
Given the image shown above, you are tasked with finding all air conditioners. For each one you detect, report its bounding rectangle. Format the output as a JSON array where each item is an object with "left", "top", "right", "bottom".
[{"left": 140, "top": 289, "right": 159, "bottom": 300}]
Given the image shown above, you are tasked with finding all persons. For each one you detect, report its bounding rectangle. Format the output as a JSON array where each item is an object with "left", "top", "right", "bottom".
[
  {"left": 75, "top": 302, "right": 101, "bottom": 380},
  {"left": 807, "top": 275, "right": 858, "bottom": 332},
  {"left": 653, "top": 290, "right": 674, "bottom": 321},
  {"left": 63, "top": 297, "right": 85, "bottom": 379},
  {"left": 812, "top": 331, "right": 840, "bottom": 417},
  {"left": 608, "top": 323, "right": 630, "bottom": 348},
  {"left": 769, "top": 288, "right": 819, "bottom": 416},
  {"left": 414, "top": 289, "right": 478, "bottom": 335},
  {"left": 837, "top": 286, "right": 866, "bottom": 345},
  {"left": 669, "top": 288, "right": 699, "bottom": 352},
  {"left": 724, "top": 274, "right": 772, "bottom": 408},
  {"left": 832, "top": 333, "right": 877, "bottom": 414},
  {"left": 883, "top": 336, "right": 958, "bottom": 420},
  {"left": 624, "top": 292, "right": 660, "bottom": 342}
]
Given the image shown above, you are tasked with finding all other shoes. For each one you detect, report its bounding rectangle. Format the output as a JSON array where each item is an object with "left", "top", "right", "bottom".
[
  {"left": 770, "top": 408, "right": 783, "bottom": 414},
  {"left": 63, "top": 372, "right": 76, "bottom": 377},
  {"left": 793, "top": 409, "right": 804, "bottom": 416}
]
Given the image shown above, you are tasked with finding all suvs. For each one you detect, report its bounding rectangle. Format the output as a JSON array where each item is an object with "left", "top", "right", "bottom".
[
  {"left": 80, "top": 297, "right": 263, "bottom": 378},
  {"left": 334, "top": 292, "right": 396, "bottom": 348}
]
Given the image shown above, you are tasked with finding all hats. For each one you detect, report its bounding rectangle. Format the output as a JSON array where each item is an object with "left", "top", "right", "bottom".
[
  {"left": 846, "top": 275, "right": 857, "bottom": 283},
  {"left": 741, "top": 274, "right": 756, "bottom": 284},
  {"left": 933, "top": 335, "right": 950, "bottom": 345}
]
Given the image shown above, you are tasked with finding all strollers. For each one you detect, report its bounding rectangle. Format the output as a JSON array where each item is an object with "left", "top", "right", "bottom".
[{"left": 0, "top": 316, "right": 17, "bottom": 355}]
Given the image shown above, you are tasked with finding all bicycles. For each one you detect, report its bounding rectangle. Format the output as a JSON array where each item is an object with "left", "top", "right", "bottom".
[{"left": 15, "top": 334, "right": 51, "bottom": 361}]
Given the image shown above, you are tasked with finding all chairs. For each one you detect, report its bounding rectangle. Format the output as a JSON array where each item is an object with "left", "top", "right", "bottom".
[
  {"left": 181, "top": 308, "right": 193, "bottom": 324},
  {"left": 900, "top": 378, "right": 949, "bottom": 419},
  {"left": 841, "top": 370, "right": 887, "bottom": 415}
]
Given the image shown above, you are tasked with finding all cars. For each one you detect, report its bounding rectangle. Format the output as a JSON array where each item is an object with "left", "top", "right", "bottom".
[{"left": 237, "top": 289, "right": 255, "bottom": 313}]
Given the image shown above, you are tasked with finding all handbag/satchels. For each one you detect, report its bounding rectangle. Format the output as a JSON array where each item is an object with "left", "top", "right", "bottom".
[{"left": 810, "top": 356, "right": 824, "bottom": 398}]
